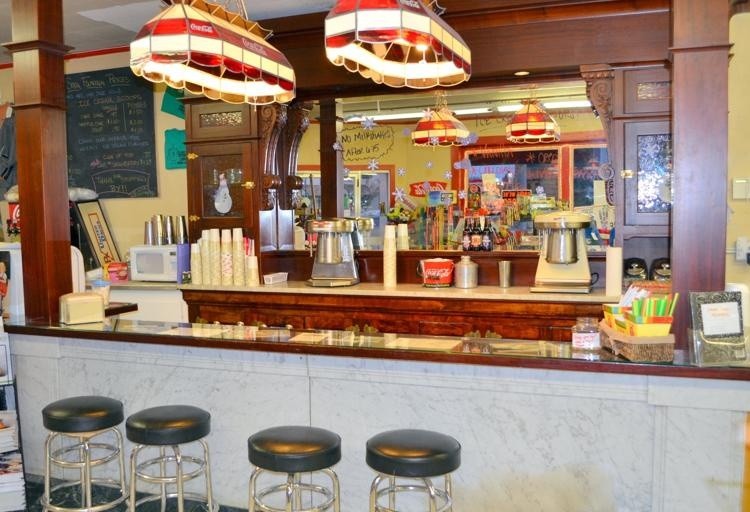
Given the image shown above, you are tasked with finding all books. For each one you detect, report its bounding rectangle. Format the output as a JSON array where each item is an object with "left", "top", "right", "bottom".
[{"left": 686, "top": 289, "right": 748, "bottom": 370}]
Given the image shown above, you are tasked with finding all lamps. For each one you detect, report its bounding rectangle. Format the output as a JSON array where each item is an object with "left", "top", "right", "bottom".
[
  {"left": 411, "top": 91, "right": 479, "bottom": 149},
  {"left": 324, "top": 0, "right": 472, "bottom": 90},
  {"left": 505, "top": 85, "right": 562, "bottom": 145},
  {"left": 130, "top": 0, "right": 297, "bottom": 106}
]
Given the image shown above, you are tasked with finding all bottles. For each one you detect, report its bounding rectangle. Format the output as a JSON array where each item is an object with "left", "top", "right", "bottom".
[
  {"left": 571, "top": 315, "right": 602, "bottom": 352},
  {"left": 453, "top": 256, "right": 479, "bottom": 289},
  {"left": 462, "top": 212, "right": 493, "bottom": 252}
]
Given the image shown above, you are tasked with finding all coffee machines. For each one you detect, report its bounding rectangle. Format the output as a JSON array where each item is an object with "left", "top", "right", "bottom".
[
  {"left": 532, "top": 212, "right": 595, "bottom": 294},
  {"left": 302, "top": 218, "right": 361, "bottom": 286}
]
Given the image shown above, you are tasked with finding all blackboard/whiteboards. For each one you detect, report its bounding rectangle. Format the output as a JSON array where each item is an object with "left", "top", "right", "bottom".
[
  {"left": 465, "top": 145, "right": 616, "bottom": 211},
  {"left": 14, "top": 66, "right": 158, "bottom": 198}
]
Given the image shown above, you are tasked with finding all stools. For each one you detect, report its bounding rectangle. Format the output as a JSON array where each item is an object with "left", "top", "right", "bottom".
[
  {"left": 126, "top": 405, "right": 219, "bottom": 512},
  {"left": 248, "top": 425, "right": 342, "bottom": 512},
  {"left": 38, "top": 396, "right": 130, "bottom": 512},
  {"left": 366, "top": 429, "right": 461, "bottom": 512}
]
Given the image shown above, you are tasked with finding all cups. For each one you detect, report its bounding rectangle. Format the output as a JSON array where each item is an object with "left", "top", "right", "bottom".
[
  {"left": 383, "top": 225, "right": 399, "bottom": 290},
  {"left": 190, "top": 228, "right": 261, "bottom": 290},
  {"left": 416, "top": 256, "right": 454, "bottom": 289},
  {"left": 497, "top": 259, "right": 514, "bottom": 289},
  {"left": 398, "top": 223, "right": 410, "bottom": 252},
  {"left": 143, "top": 214, "right": 186, "bottom": 244}
]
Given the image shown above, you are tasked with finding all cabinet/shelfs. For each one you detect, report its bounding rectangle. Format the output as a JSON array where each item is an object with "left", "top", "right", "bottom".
[{"left": 612, "top": 116, "right": 671, "bottom": 248}]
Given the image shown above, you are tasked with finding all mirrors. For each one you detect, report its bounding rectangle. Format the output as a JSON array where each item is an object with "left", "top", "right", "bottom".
[{"left": 288, "top": 78, "right": 614, "bottom": 251}]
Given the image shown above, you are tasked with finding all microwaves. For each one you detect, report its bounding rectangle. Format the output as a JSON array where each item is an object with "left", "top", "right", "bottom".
[{"left": 128, "top": 246, "right": 180, "bottom": 283}]
